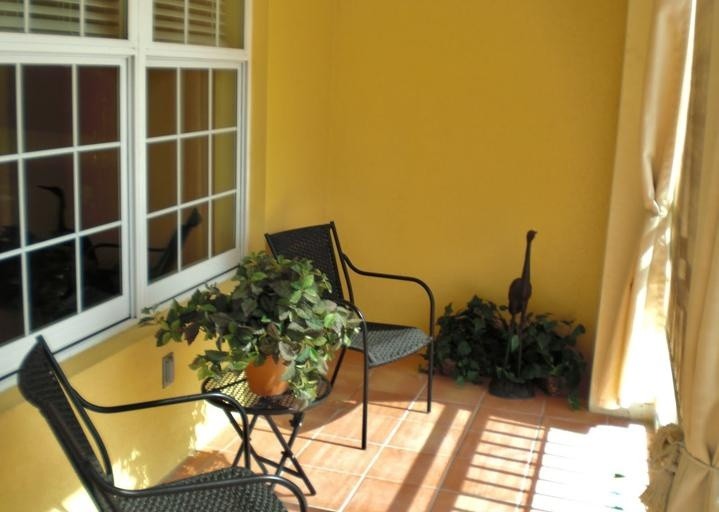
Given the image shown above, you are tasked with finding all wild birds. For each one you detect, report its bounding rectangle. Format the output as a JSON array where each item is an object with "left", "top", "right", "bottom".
[
  {"left": 500, "top": 230, "right": 538, "bottom": 379},
  {"left": 35, "top": 184, "right": 92, "bottom": 243}
]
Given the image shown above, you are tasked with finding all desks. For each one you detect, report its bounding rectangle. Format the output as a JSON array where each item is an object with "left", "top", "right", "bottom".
[{"left": 199, "top": 368, "right": 332, "bottom": 500}]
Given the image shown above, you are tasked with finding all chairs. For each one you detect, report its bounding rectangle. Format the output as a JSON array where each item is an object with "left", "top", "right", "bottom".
[
  {"left": 1, "top": 182, "right": 203, "bottom": 324},
  {"left": 14, "top": 332, "right": 315, "bottom": 511},
  {"left": 264, "top": 220, "right": 439, "bottom": 451}
]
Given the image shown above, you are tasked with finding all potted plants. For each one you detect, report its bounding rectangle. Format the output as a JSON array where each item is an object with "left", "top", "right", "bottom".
[{"left": 138, "top": 245, "right": 366, "bottom": 405}]
